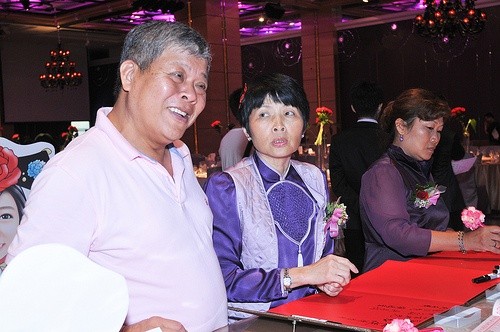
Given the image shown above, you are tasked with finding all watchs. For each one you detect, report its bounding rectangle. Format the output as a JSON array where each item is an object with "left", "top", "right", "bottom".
[{"left": 283, "top": 267, "right": 293, "bottom": 293}]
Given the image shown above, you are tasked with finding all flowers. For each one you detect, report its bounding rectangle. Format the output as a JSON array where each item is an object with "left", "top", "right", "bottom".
[
  {"left": 451, "top": 107, "right": 472, "bottom": 131},
  {"left": 61, "top": 126, "right": 78, "bottom": 143},
  {"left": 383, "top": 318, "right": 418, "bottom": 332},
  {"left": 314, "top": 106, "right": 334, "bottom": 146},
  {"left": 414, "top": 181, "right": 447, "bottom": 209},
  {"left": 320, "top": 197, "right": 349, "bottom": 226},
  {"left": 12, "top": 133, "right": 19, "bottom": 143},
  {"left": 210, "top": 120, "right": 223, "bottom": 135},
  {"left": 461, "top": 206, "right": 485, "bottom": 231}
]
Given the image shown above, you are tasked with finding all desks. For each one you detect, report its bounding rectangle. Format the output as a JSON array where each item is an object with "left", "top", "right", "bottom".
[
  {"left": 212, "top": 283, "right": 500, "bottom": 332},
  {"left": 473, "top": 161, "right": 500, "bottom": 215}
]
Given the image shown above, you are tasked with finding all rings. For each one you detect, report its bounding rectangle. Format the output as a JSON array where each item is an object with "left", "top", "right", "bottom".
[{"left": 494, "top": 241, "right": 497, "bottom": 247}]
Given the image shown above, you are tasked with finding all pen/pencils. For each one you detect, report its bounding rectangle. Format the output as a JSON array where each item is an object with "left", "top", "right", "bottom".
[{"left": 477, "top": 219, "right": 485, "bottom": 227}]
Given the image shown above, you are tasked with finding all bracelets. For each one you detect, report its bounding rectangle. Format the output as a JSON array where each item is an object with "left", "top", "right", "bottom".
[
  {"left": 460, "top": 231, "right": 466, "bottom": 253},
  {"left": 458, "top": 231, "right": 461, "bottom": 252}
]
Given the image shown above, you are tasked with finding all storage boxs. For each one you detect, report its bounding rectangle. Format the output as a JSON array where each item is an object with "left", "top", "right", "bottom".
[
  {"left": 485, "top": 284, "right": 500, "bottom": 302},
  {"left": 434, "top": 305, "right": 482, "bottom": 329}
]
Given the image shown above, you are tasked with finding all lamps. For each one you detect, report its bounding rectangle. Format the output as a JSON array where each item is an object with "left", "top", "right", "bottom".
[
  {"left": 415, "top": 0, "right": 487, "bottom": 48},
  {"left": 40, "top": 25, "right": 83, "bottom": 95}
]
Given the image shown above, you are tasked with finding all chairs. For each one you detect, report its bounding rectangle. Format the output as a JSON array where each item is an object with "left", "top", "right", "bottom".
[{"left": 451, "top": 156, "right": 478, "bottom": 209}]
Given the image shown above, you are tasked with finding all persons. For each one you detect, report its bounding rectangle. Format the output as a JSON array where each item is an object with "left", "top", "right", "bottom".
[
  {"left": 212, "top": 73, "right": 500, "bottom": 322},
  {"left": 8, "top": 18, "right": 229, "bottom": 332}
]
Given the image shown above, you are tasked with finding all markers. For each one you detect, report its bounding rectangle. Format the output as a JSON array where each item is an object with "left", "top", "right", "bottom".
[{"left": 472, "top": 273, "right": 499, "bottom": 283}]
